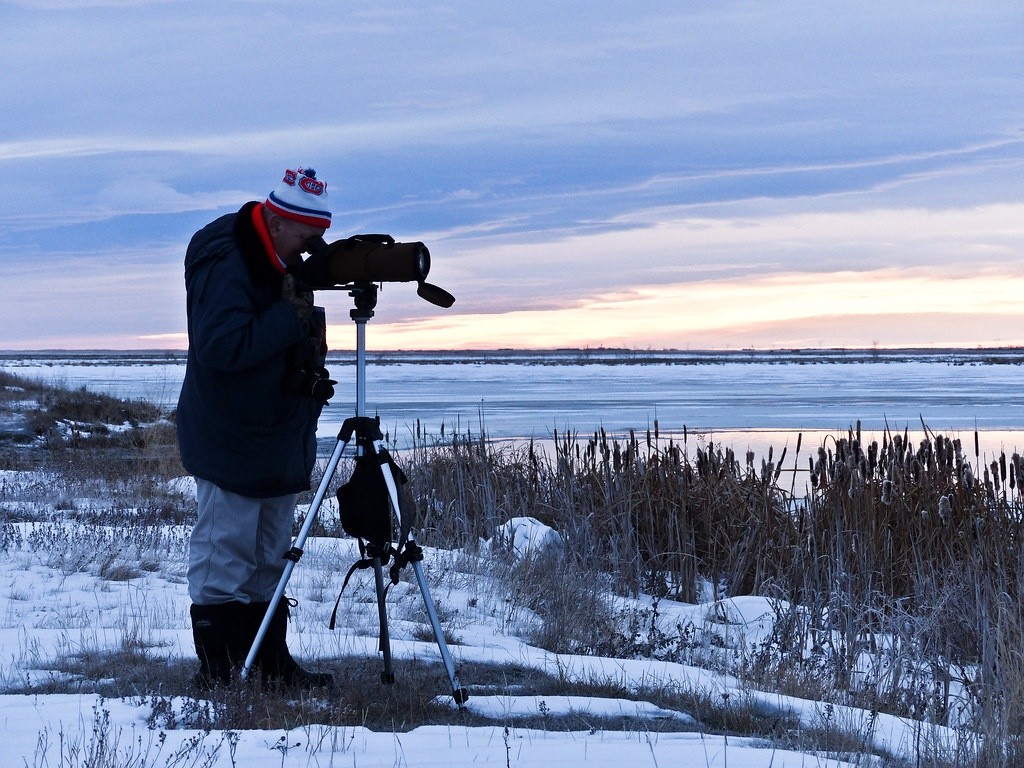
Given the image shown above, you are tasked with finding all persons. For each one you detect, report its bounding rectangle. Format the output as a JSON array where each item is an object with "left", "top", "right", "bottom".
[{"left": 174, "top": 168, "right": 339, "bottom": 691}]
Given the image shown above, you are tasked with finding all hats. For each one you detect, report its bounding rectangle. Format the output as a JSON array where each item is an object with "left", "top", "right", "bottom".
[{"left": 266, "top": 166, "right": 332, "bottom": 228}]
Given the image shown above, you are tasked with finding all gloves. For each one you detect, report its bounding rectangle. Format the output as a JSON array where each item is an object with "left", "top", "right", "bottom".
[{"left": 282, "top": 268, "right": 314, "bottom": 327}]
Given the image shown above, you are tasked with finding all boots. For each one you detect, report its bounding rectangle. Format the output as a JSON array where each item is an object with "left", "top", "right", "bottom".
[
  {"left": 246, "top": 595, "right": 334, "bottom": 691},
  {"left": 190, "top": 600, "right": 275, "bottom": 697}
]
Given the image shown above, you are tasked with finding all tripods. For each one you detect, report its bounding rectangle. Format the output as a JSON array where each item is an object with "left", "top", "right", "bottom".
[{"left": 240, "top": 280, "right": 471, "bottom": 712}]
